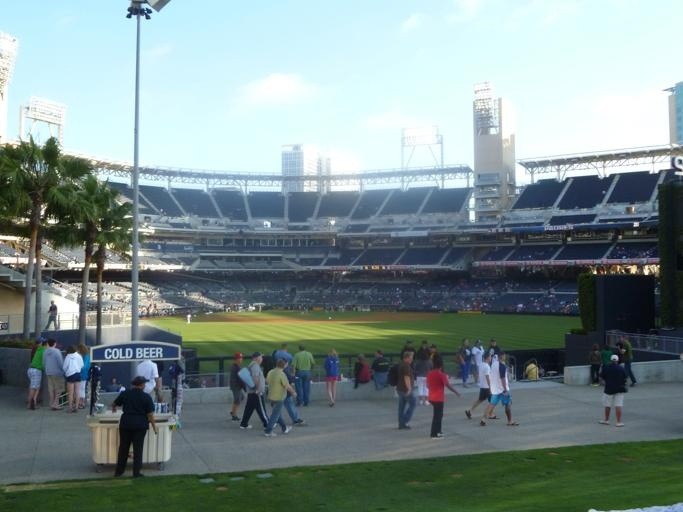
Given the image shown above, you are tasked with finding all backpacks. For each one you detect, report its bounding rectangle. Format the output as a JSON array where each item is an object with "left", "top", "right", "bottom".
[{"left": 388, "top": 364, "right": 401, "bottom": 386}]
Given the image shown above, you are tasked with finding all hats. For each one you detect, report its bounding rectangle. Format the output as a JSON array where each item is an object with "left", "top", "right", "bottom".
[
  {"left": 234, "top": 352, "right": 246, "bottom": 358},
  {"left": 131, "top": 376, "right": 149, "bottom": 385},
  {"left": 252, "top": 352, "right": 261, "bottom": 358}
]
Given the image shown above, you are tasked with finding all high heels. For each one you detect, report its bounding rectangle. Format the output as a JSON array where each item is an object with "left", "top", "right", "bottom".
[{"left": 328, "top": 403, "right": 335, "bottom": 407}]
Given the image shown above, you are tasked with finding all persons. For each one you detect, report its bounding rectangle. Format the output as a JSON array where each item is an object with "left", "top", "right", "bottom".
[
  {"left": 113, "top": 373, "right": 158, "bottom": 478},
  {"left": 135, "top": 358, "right": 163, "bottom": 404},
  {"left": 397, "top": 340, "right": 440, "bottom": 429},
  {"left": 587, "top": 332, "right": 638, "bottom": 387},
  {"left": 459, "top": 340, "right": 518, "bottom": 426},
  {"left": 41, "top": 300, "right": 59, "bottom": 331},
  {"left": 354, "top": 351, "right": 391, "bottom": 383},
  {"left": 24, "top": 337, "right": 90, "bottom": 415},
  {"left": 325, "top": 349, "right": 341, "bottom": 406},
  {"left": 262, "top": 355, "right": 297, "bottom": 438},
  {"left": 230, "top": 352, "right": 277, "bottom": 430},
  {"left": 105, "top": 376, "right": 121, "bottom": 392},
  {"left": 276, "top": 345, "right": 315, "bottom": 406},
  {"left": 0, "top": 215, "right": 661, "bottom": 321},
  {"left": 599, "top": 353, "right": 629, "bottom": 428},
  {"left": 425, "top": 355, "right": 462, "bottom": 441}
]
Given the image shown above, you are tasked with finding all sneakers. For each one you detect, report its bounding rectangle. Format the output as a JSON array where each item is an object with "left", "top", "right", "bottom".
[
  {"left": 114, "top": 473, "right": 121, "bottom": 477},
  {"left": 133, "top": 474, "right": 144, "bottom": 477},
  {"left": 600, "top": 420, "right": 609, "bottom": 424},
  {"left": 398, "top": 400, "right": 520, "bottom": 440},
  {"left": 230, "top": 401, "right": 307, "bottom": 437},
  {"left": 616, "top": 422, "right": 625, "bottom": 427},
  {"left": 611, "top": 355, "right": 618, "bottom": 361}
]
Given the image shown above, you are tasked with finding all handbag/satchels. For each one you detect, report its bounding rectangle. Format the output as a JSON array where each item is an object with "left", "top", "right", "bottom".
[{"left": 236, "top": 368, "right": 255, "bottom": 389}]
